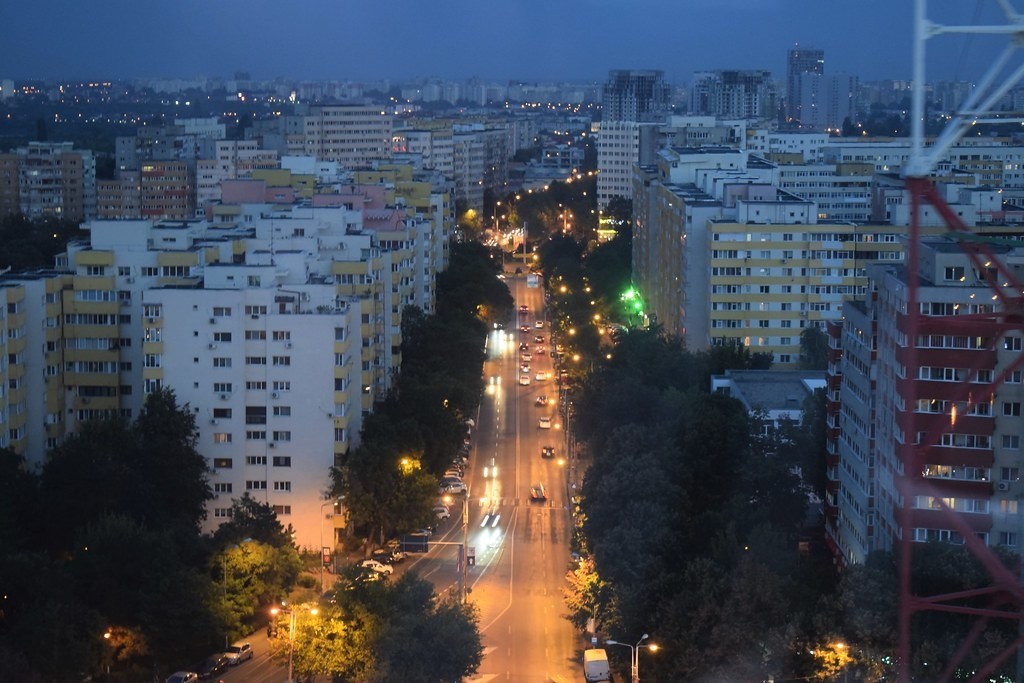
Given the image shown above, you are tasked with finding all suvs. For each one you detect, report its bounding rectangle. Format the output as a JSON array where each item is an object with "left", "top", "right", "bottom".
[{"left": 270, "top": 601, "right": 319, "bottom": 683}]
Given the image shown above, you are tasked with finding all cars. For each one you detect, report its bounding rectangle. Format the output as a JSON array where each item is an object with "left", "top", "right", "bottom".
[
  {"left": 532, "top": 270, "right": 543, "bottom": 277},
  {"left": 607, "top": 323, "right": 629, "bottom": 346},
  {"left": 514, "top": 267, "right": 522, "bottom": 273},
  {"left": 164, "top": 671, "right": 199, "bottom": 683},
  {"left": 517, "top": 304, "right": 556, "bottom": 503},
  {"left": 267, "top": 618, "right": 290, "bottom": 637},
  {"left": 195, "top": 653, "right": 229, "bottom": 679},
  {"left": 325, "top": 417, "right": 475, "bottom": 603}
]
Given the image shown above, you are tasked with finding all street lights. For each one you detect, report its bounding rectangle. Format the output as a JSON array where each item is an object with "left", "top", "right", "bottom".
[
  {"left": 97, "top": 626, "right": 116, "bottom": 673},
  {"left": 320, "top": 495, "right": 346, "bottom": 598},
  {"left": 606, "top": 634, "right": 661, "bottom": 683},
  {"left": 223, "top": 536, "right": 253, "bottom": 648}
]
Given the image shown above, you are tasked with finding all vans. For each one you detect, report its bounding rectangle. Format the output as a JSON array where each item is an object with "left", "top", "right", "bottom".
[
  {"left": 222, "top": 642, "right": 254, "bottom": 666},
  {"left": 584, "top": 648, "right": 612, "bottom": 683}
]
